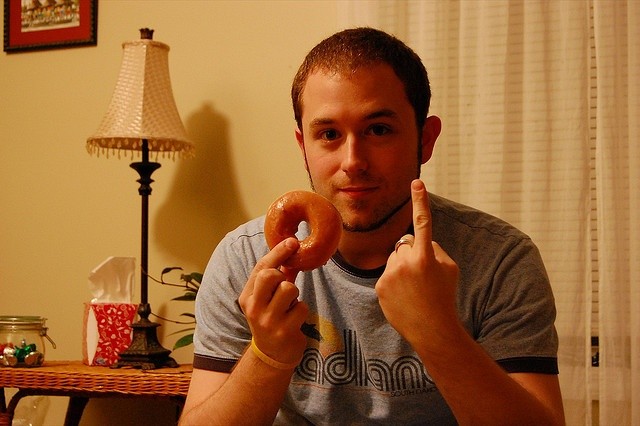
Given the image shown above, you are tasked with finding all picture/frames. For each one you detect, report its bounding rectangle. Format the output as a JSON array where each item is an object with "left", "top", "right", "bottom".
[{"left": 3, "top": 0, "right": 99, "bottom": 52}]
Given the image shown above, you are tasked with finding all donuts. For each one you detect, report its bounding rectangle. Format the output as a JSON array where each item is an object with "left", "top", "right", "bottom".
[{"left": 264, "top": 191, "right": 344, "bottom": 269}]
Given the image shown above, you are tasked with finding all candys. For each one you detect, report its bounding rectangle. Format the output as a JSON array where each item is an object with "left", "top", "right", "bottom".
[{"left": 0, "top": 343, "right": 44, "bottom": 368}]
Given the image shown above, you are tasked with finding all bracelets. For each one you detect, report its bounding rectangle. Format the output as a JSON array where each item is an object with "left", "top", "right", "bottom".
[{"left": 251, "top": 335, "right": 304, "bottom": 370}]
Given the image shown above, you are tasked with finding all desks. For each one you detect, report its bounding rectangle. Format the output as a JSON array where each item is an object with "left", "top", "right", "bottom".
[{"left": 0, "top": 362, "right": 194, "bottom": 426}]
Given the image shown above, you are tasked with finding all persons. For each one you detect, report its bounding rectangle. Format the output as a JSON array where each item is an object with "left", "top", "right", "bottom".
[{"left": 177, "top": 28, "right": 566, "bottom": 425}]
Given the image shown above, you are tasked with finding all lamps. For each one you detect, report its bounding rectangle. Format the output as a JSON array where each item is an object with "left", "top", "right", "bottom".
[{"left": 86, "top": 27, "right": 195, "bottom": 372}]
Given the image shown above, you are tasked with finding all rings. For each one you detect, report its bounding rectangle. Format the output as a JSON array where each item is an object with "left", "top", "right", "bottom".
[{"left": 395, "top": 238, "right": 414, "bottom": 252}]
